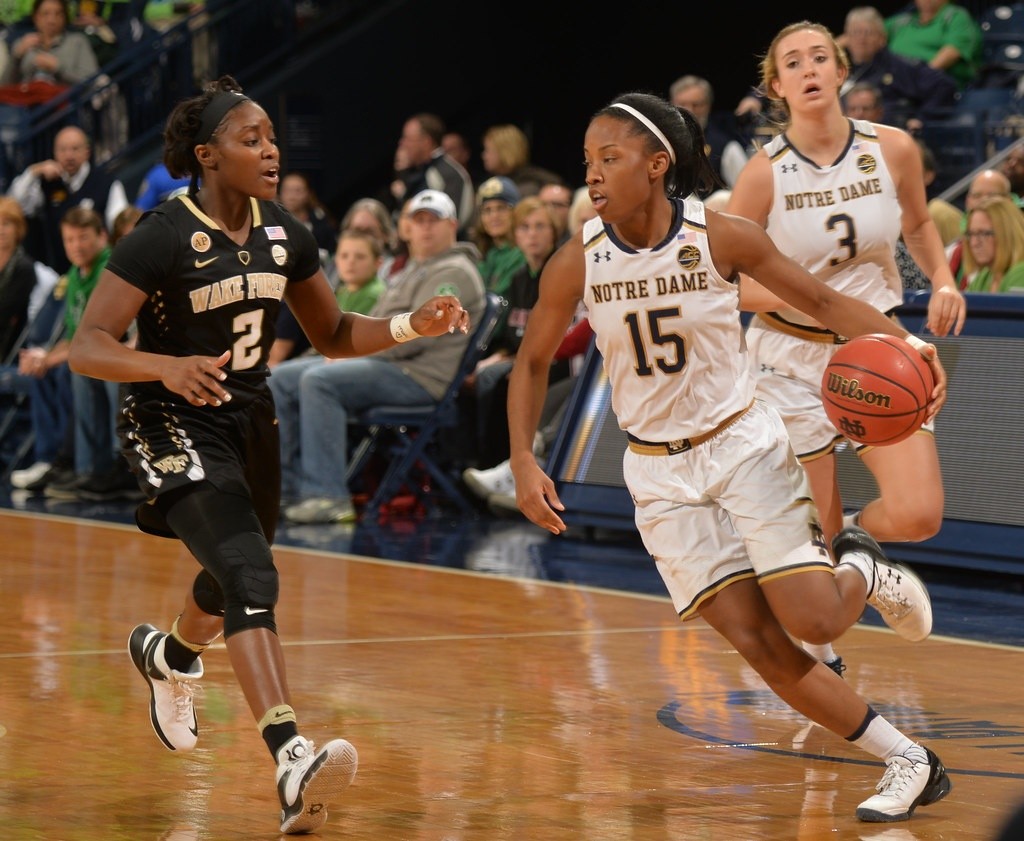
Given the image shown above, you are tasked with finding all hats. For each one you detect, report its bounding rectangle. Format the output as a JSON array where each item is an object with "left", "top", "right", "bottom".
[
  {"left": 479, "top": 177, "right": 520, "bottom": 205},
  {"left": 409, "top": 190, "right": 455, "bottom": 218}
]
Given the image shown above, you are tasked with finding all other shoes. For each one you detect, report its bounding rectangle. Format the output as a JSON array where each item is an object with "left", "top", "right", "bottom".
[
  {"left": 463, "top": 457, "right": 549, "bottom": 518},
  {"left": 354, "top": 471, "right": 431, "bottom": 515},
  {"left": 843, "top": 511, "right": 859, "bottom": 527},
  {"left": 824, "top": 657, "right": 842, "bottom": 676},
  {"left": 11, "top": 463, "right": 148, "bottom": 500}
]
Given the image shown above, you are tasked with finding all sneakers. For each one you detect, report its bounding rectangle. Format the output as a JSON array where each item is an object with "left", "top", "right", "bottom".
[
  {"left": 128, "top": 624, "right": 204, "bottom": 752},
  {"left": 286, "top": 498, "right": 356, "bottom": 522},
  {"left": 277, "top": 735, "right": 358, "bottom": 833},
  {"left": 831, "top": 526, "right": 933, "bottom": 642},
  {"left": 855, "top": 746, "right": 952, "bottom": 821},
  {"left": 777, "top": 723, "right": 850, "bottom": 751}
]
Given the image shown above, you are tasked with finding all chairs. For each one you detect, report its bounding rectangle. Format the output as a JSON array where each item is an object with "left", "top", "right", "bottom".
[
  {"left": 345, "top": 295, "right": 507, "bottom": 527},
  {"left": 1, "top": 288, "right": 73, "bottom": 471}
]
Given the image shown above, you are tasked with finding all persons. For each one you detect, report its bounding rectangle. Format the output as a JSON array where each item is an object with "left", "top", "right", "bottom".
[
  {"left": 508, "top": 92, "right": 953, "bottom": 824},
  {"left": 267, "top": 188, "right": 489, "bottom": 524},
  {"left": 950, "top": 198, "right": 1023, "bottom": 298},
  {"left": 65, "top": 75, "right": 469, "bottom": 835},
  {"left": 1, "top": 0, "right": 477, "bottom": 524},
  {"left": 463, "top": 0, "right": 1023, "bottom": 517},
  {"left": 722, "top": 20, "right": 964, "bottom": 681}
]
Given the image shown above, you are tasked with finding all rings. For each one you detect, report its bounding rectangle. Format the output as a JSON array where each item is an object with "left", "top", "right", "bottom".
[{"left": 455, "top": 306, "right": 463, "bottom": 312}]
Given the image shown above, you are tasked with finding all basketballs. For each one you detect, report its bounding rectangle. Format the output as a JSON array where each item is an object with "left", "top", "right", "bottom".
[{"left": 820, "top": 333, "right": 934, "bottom": 447}]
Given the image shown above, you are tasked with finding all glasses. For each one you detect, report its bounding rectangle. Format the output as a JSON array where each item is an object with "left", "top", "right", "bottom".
[
  {"left": 848, "top": 105, "right": 873, "bottom": 112},
  {"left": 542, "top": 202, "right": 568, "bottom": 208},
  {"left": 965, "top": 230, "right": 994, "bottom": 240}
]
